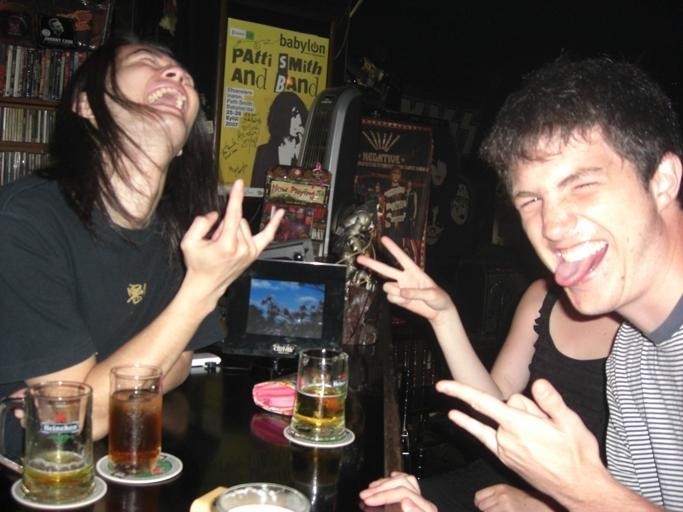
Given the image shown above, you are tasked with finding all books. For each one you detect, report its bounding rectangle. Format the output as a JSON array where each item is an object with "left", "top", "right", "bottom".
[{"left": 0, "top": 0, "right": 114, "bottom": 185}]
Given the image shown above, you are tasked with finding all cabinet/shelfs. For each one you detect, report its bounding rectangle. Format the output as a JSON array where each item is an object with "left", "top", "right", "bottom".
[{"left": 2, "top": 39, "right": 91, "bottom": 185}]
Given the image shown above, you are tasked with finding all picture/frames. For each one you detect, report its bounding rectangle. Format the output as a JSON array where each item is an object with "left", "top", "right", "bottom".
[{"left": 224, "top": 258, "right": 347, "bottom": 357}]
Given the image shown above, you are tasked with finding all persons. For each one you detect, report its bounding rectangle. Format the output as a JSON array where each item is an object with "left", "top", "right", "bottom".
[
  {"left": 355, "top": 236, "right": 625, "bottom": 512},
  {"left": 359, "top": 67, "right": 683, "bottom": 511},
  {"left": 406, "top": 178, "right": 418, "bottom": 231},
  {"left": 383, "top": 165, "right": 408, "bottom": 233},
  {"left": 250, "top": 92, "right": 308, "bottom": 187},
  {"left": 0, "top": 32, "right": 286, "bottom": 464}
]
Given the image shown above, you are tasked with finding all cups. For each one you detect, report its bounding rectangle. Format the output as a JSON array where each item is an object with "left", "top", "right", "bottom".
[
  {"left": 0, "top": 382, "right": 95, "bottom": 504},
  {"left": 107, "top": 364, "right": 165, "bottom": 473},
  {"left": 213, "top": 482, "right": 313, "bottom": 512},
  {"left": 291, "top": 345, "right": 348, "bottom": 443}
]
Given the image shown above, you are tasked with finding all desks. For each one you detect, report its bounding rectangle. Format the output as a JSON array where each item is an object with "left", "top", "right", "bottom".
[{"left": 0, "top": 357, "right": 406, "bottom": 512}]
